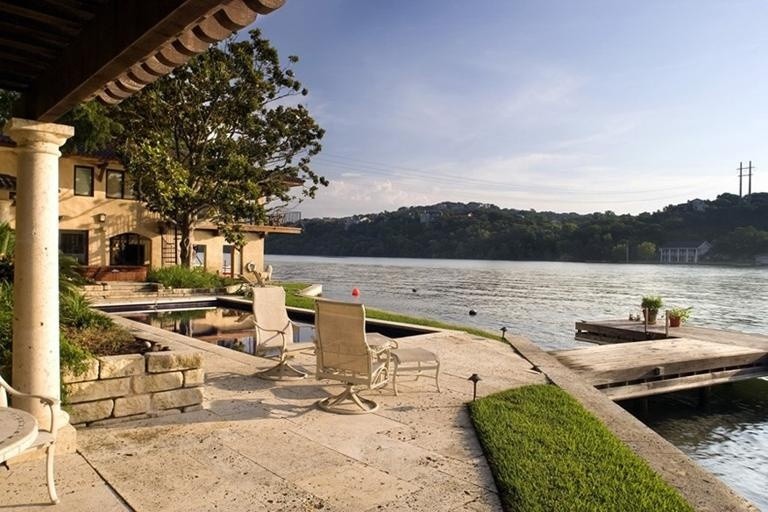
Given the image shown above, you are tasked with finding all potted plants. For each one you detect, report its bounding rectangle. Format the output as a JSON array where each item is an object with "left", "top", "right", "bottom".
[
  {"left": 670, "top": 306, "right": 694, "bottom": 327},
  {"left": 641, "top": 296, "right": 663, "bottom": 324}
]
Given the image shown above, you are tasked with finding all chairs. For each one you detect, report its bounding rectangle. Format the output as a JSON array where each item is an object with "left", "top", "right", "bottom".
[
  {"left": 250, "top": 286, "right": 316, "bottom": 381},
  {"left": 0, "top": 377, "right": 61, "bottom": 506},
  {"left": 314, "top": 297, "right": 391, "bottom": 414}
]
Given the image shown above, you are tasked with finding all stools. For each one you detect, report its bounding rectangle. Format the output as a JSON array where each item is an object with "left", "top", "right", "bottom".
[
  {"left": 392, "top": 346, "right": 442, "bottom": 396},
  {"left": 366, "top": 332, "right": 399, "bottom": 390}
]
